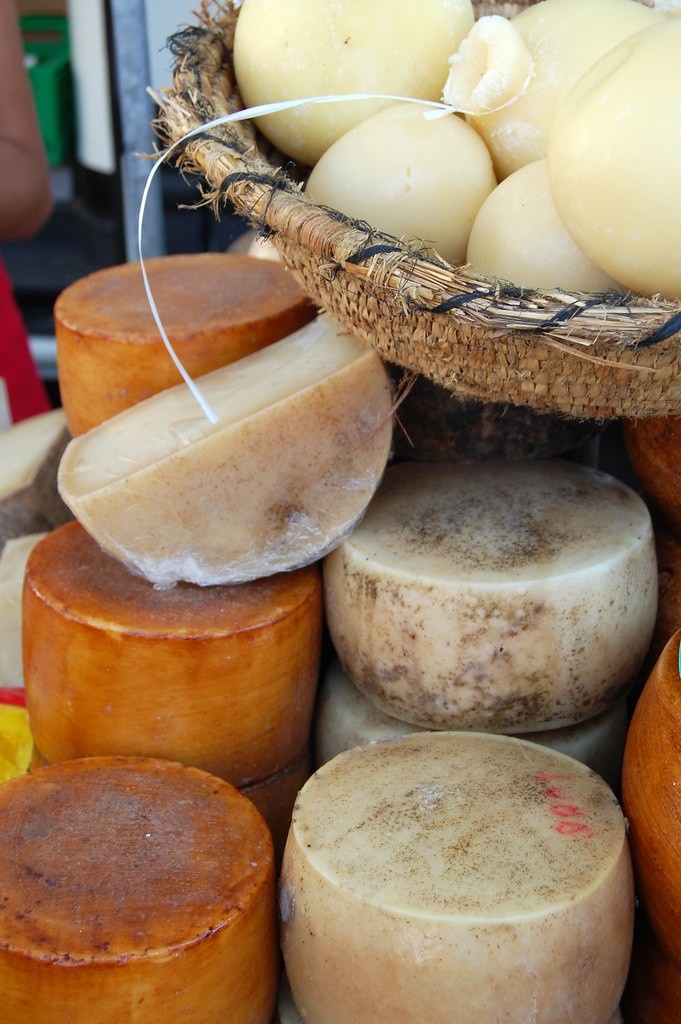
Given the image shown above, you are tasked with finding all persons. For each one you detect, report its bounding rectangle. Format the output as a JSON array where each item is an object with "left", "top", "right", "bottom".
[{"left": 0, "top": 0, "right": 53, "bottom": 429}]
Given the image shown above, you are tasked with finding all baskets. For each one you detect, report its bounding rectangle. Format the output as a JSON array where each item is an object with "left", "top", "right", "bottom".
[{"left": 147, "top": 0, "right": 681, "bottom": 418}]
